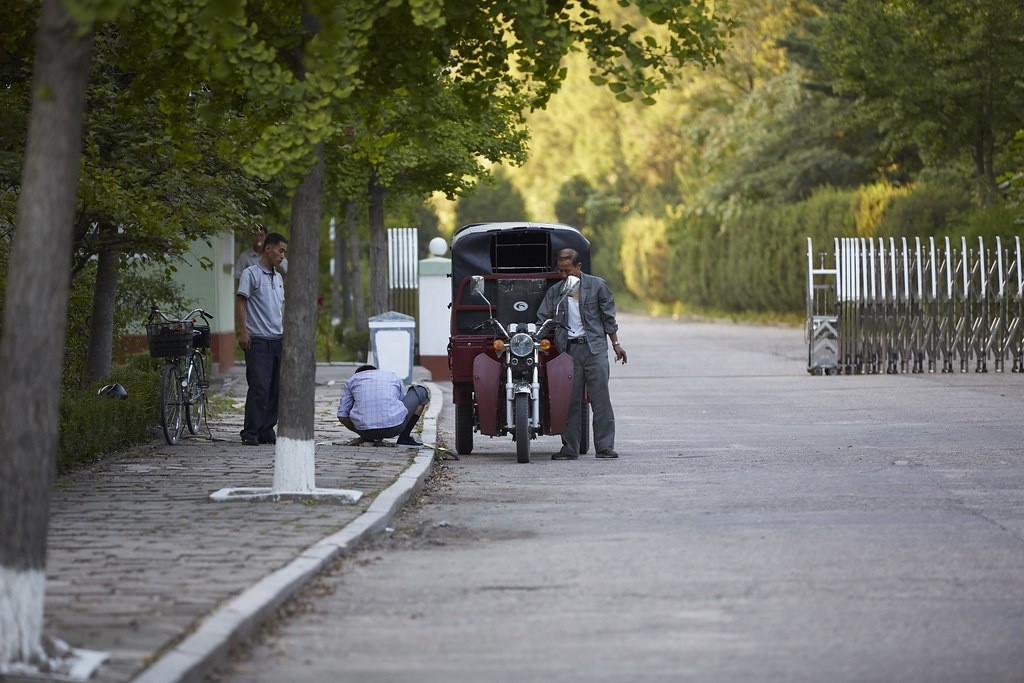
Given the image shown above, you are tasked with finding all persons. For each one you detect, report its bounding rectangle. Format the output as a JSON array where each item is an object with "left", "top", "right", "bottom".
[
  {"left": 337, "top": 365, "right": 431, "bottom": 449},
  {"left": 235, "top": 225, "right": 287, "bottom": 446},
  {"left": 611, "top": 342, "right": 620, "bottom": 345},
  {"left": 533, "top": 248, "right": 627, "bottom": 459}
]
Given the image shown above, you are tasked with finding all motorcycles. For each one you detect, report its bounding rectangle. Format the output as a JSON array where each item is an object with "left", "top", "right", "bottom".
[{"left": 445, "top": 223, "right": 592, "bottom": 465}]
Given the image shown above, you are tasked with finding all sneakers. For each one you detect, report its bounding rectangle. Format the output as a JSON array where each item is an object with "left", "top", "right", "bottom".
[
  {"left": 359, "top": 438, "right": 376, "bottom": 447},
  {"left": 397, "top": 436, "right": 424, "bottom": 449}
]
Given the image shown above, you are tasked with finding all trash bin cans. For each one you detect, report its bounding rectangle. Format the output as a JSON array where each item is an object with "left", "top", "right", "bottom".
[{"left": 368, "top": 310, "right": 418, "bottom": 386}]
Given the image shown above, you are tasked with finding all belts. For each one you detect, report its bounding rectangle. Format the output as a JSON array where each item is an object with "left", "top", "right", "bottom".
[
  {"left": 252, "top": 339, "right": 278, "bottom": 344},
  {"left": 570, "top": 337, "right": 586, "bottom": 344}
]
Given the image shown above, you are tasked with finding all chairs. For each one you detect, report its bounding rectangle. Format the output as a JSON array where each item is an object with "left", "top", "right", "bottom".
[{"left": 493, "top": 277, "right": 546, "bottom": 337}]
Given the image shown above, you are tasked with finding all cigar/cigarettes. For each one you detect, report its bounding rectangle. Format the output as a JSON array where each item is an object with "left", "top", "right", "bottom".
[{"left": 621, "top": 361, "right": 625, "bottom": 363}]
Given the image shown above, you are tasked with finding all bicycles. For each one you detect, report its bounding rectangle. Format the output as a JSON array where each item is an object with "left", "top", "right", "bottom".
[{"left": 146, "top": 305, "right": 214, "bottom": 445}]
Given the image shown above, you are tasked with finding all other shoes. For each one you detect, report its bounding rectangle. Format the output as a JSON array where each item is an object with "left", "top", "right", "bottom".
[
  {"left": 595, "top": 449, "right": 618, "bottom": 458},
  {"left": 258, "top": 435, "right": 276, "bottom": 444},
  {"left": 241, "top": 437, "right": 258, "bottom": 446},
  {"left": 552, "top": 452, "right": 580, "bottom": 460}
]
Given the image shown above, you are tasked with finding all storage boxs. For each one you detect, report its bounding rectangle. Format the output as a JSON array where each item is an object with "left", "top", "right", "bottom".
[{"left": 193, "top": 325, "right": 211, "bottom": 348}]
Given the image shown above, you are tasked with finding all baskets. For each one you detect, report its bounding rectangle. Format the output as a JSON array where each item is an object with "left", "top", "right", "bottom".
[{"left": 146, "top": 322, "right": 194, "bottom": 358}]
